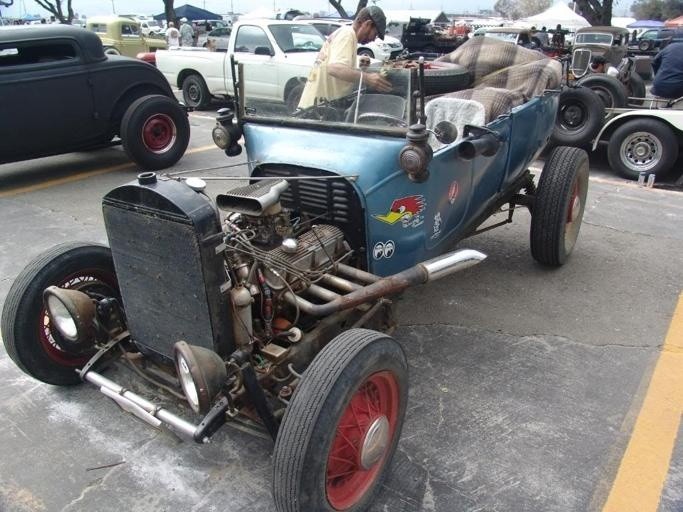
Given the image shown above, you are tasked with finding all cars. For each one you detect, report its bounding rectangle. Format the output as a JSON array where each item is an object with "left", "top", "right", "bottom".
[
  {"left": 1, "top": 25, "right": 191, "bottom": 170},
  {"left": 384, "top": 26, "right": 682, "bottom": 182},
  {"left": 0, "top": 36, "right": 590, "bottom": 508}
]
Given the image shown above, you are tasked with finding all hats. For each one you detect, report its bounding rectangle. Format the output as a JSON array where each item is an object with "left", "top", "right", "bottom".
[{"left": 368, "top": 6, "right": 386, "bottom": 41}]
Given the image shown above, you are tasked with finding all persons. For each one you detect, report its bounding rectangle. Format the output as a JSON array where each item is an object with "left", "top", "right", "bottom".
[
  {"left": 165, "top": 17, "right": 199, "bottom": 48},
  {"left": 650, "top": 31, "right": 682, "bottom": 98},
  {"left": 530, "top": 25, "right": 565, "bottom": 49},
  {"left": 296, "top": 4, "right": 393, "bottom": 113}
]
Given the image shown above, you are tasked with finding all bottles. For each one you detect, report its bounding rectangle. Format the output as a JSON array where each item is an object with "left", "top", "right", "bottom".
[{"left": 638, "top": 171, "right": 656, "bottom": 187}]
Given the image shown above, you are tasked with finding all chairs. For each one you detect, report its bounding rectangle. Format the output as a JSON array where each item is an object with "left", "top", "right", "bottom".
[{"left": 348, "top": 92, "right": 486, "bottom": 152}]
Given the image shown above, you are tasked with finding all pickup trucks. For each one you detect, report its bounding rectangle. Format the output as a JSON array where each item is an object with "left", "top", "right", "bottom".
[{"left": 156, "top": 3, "right": 391, "bottom": 115}]
